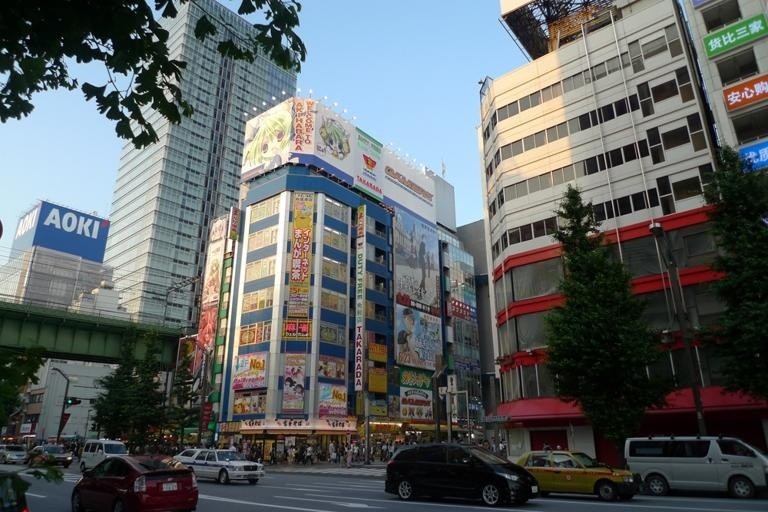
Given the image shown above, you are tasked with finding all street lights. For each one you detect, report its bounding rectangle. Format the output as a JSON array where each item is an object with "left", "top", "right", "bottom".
[
  {"left": 49, "top": 366, "right": 71, "bottom": 445},
  {"left": 450, "top": 390, "right": 471, "bottom": 444},
  {"left": 648, "top": 222, "right": 706, "bottom": 435}
]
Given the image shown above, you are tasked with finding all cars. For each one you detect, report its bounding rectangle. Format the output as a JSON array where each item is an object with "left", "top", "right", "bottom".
[
  {"left": 70, "top": 455, "right": 199, "bottom": 512},
  {"left": 383, "top": 442, "right": 540, "bottom": 506},
  {"left": 26, "top": 445, "right": 73, "bottom": 469},
  {"left": 516, "top": 449, "right": 642, "bottom": 502},
  {"left": 0, "top": 444, "right": 29, "bottom": 464},
  {"left": 0, "top": 471, "right": 29, "bottom": 512}
]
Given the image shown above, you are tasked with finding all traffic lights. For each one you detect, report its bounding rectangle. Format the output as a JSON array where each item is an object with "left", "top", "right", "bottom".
[{"left": 66, "top": 399, "right": 82, "bottom": 406}]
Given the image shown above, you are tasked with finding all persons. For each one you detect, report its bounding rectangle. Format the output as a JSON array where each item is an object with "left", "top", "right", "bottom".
[
  {"left": 396, "top": 306, "right": 437, "bottom": 366},
  {"left": 0, "top": 436, "right": 561, "bottom": 469},
  {"left": 284, "top": 374, "right": 306, "bottom": 399},
  {"left": 416, "top": 232, "right": 430, "bottom": 294}
]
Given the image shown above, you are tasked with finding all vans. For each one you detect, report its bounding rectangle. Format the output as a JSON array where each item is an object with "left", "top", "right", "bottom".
[
  {"left": 79, "top": 439, "right": 128, "bottom": 474},
  {"left": 624, "top": 436, "right": 767, "bottom": 499}
]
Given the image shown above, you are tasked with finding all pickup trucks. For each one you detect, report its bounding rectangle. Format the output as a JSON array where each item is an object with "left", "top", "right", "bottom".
[{"left": 172, "top": 449, "right": 265, "bottom": 485}]
